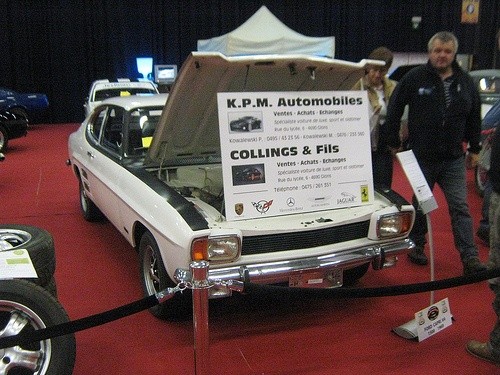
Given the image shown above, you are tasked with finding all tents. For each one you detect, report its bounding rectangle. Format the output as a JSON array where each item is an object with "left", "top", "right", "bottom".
[{"left": 197, "top": 5, "right": 334, "bottom": 58}]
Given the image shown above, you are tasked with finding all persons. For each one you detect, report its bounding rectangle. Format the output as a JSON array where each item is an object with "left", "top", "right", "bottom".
[
  {"left": 383, "top": 33, "right": 486, "bottom": 276},
  {"left": 350, "top": 47, "right": 403, "bottom": 191},
  {"left": 465, "top": 123, "right": 500, "bottom": 366}
]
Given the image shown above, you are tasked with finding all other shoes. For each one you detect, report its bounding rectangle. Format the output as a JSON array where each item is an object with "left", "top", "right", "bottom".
[
  {"left": 466, "top": 340, "right": 500, "bottom": 363},
  {"left": 409, "top": 249, "right": 428, "bottom": 265},
  {"left": 464, "top": 259, "right": 485, "bottom": 274}
]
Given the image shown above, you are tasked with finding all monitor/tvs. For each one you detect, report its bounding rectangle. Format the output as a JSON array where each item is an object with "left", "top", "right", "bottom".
[{"left": 154, "top": 65, "right": 177, "bottom": 84}]
